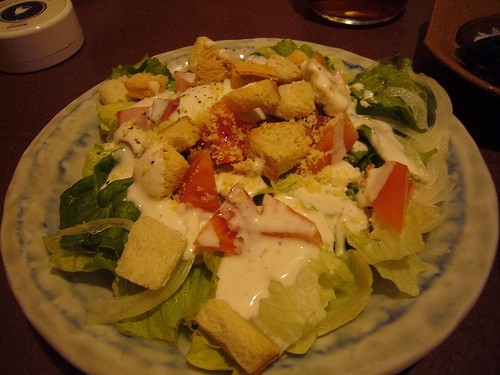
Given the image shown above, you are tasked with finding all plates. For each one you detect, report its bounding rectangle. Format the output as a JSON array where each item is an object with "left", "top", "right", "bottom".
[
  {"left": 0, "top": 38, "right": 500, "bottom": 375},
  {"left": 422, "top": 0, "right": 500, "bottom": 96}
]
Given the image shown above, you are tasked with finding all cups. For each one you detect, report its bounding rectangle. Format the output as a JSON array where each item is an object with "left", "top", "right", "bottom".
[{"left": 312, "top": 0, "right": 405, "bottom": 26}]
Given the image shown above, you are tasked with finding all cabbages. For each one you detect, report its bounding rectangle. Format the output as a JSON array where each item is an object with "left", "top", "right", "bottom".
[{"left": 42, "top": 37, "right": 453, "bottom": 370}]
